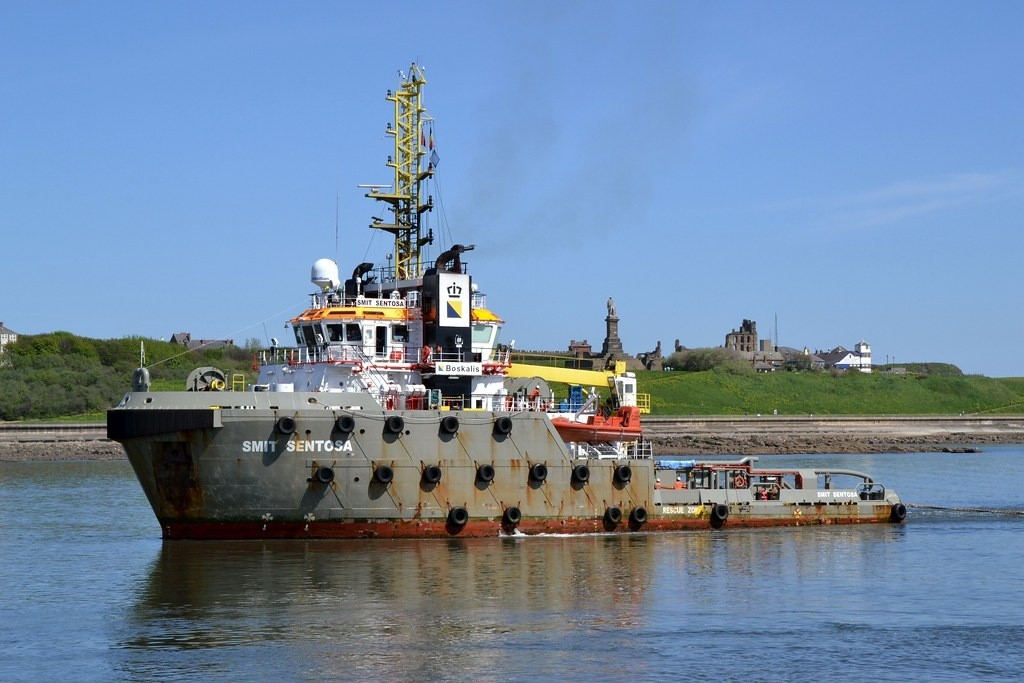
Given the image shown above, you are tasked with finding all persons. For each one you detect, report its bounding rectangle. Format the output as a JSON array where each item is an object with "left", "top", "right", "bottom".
[
  {"left": 607, "top": 298, "right": 615, "bottom": 316},
  {"left": 673, "top": 477, "right": 682, "bottom": 490},
  {"left": 656, "top": 479, "right": 661, "bottom": 490},
  {"left": 530, "top": 385, "right": 539, "bottom": 408}
]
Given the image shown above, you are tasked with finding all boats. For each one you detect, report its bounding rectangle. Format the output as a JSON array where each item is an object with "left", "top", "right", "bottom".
[{"left": 106, "top": 60, "right": 905, "bottom": 540}]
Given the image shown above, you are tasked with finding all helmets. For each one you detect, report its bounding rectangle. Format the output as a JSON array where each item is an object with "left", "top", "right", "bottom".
[
  {"left": 677, "top": 476, "right": 681, "bottom": 480},
  {"left": 535, "top": 384, "right": 540, "bottom": 388},
  {"left": 656, "top": 478, "right": 660, "bottom": 481}
]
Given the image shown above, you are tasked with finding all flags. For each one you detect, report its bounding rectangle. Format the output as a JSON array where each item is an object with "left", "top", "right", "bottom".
[
  {"left": 421, "top": 130, "right": 426, "bottom": 146},
  {"left": 429, "top": 122, "right": 434, "bottom": 151}
]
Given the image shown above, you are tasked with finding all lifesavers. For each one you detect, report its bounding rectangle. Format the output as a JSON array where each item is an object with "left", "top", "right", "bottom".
[
  {"left": 494, "top": 416, "right": 513, "bottom": 434},
  {"left": 476, "top": 464, "right": 495, "bottom": 483},
  {"left": 441, "top": 415, "right": 460, "bottom": 434},
  {"left": 712, "top": 504, "right": 729, "bottom": 520},
  {"left": 316, "top": 465, "right": 335, "bottom": 483},
  {"left": 374, "top": 464, "right": 394, "bottom": 484},
  {"left": 614, "top": 464, "right": 633, "bottom": 485},
  {"left": 423, "top": 464, "right": 443, "bottom": 484},
  {"left": 892, "top": 503, "right": 907, "bottom": 521},
  {"left": 503, "top": 506, "right": 522, "bottom": 525},
  {"left": 630, "top": 505, "right": 648, "bottom": 525},
  {"left": 734, "top": 475, "right": 745, "bottom": 488},
  {"left": 277, "top": 415, "right": 297, "bottom": 435},
  {"left": 529, "top": 463, "right": 549, "bottom": 482},
  {"left": 335, "top": 415, "right": 356, "bottom": 433},
  {"left": 572, "top": 464, "right": 591, "bottom": 483},
  {"left": 385, "top": 415, "right": 405, "bottom": 434},
  {"left": 604, "top": 506, "right": 622, "bottom": 524},
  {"left": 447, "top": 505, "right": 469, "bottom": 529},
  {"left": 421, "top": 346, "right": 432, "bottom": 361}
]
